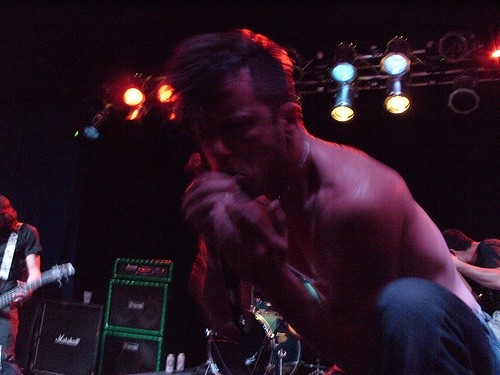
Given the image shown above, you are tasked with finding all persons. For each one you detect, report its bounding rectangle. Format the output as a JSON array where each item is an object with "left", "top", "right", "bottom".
[
  {"left": 440, "top": 229, "right": 500, "bottom": 342},
  {"left": 0, "top": 195, "right": 43, "bottom": 368},
  {"left": 179, "top": 144, "right": 255, "bottom": 324},
  {"left": 166, "top": 29, "right": 500, "bottom": 375}
]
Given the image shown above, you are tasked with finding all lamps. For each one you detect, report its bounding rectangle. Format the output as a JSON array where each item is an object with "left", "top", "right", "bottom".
[
  {"left": 329, "top": 41, "right": 357, "bottom": 84},
  {"left": 329, "top": 82, "right": 358, "bottom": 123},
  {"left": 84, "top": 102, "right": 116, "bottom": 139},
  {"left": 123, "top": 78, "right": 146, "bottom": 133},
  {"left": 436, "top": 28, "right": 474, "bottom": 64},
  {"left": 383, "top": 75, "right": 414, "bottom": 115},
  {"left": 447, "top": 73, "right": 479, "bottom": 115},
  {"left": 380, "top": 36, "right": 412, "bottom": 78}
]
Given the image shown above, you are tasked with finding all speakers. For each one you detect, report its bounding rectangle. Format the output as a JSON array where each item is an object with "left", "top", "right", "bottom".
[{"left": 11, "top": 278, "right": 170, "bottom": 375}]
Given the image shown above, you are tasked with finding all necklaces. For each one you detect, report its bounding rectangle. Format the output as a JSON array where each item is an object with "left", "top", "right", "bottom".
[{"left": 272, "top": 141, "right": 310, "bottom": 205}]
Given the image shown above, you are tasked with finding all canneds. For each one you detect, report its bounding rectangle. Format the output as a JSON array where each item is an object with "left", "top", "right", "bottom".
[
  {"left": 177, "top": 353, "right": 185, "bottom": 371},
  {"left": 165, "top": 354, "right": 175, "bottom": 373}
]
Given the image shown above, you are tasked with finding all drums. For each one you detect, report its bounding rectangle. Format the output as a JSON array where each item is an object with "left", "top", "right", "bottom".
[{"left": 206, "top": 298, "right": 301, "bottom": 375}]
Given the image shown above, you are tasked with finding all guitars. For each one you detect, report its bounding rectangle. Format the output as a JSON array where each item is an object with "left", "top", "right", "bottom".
[{"left": 0, "top": 263, "right": 75, "bottom": 310}]
